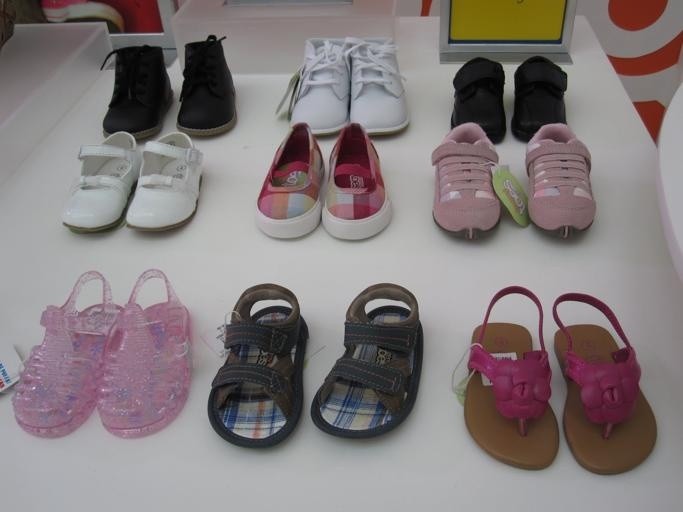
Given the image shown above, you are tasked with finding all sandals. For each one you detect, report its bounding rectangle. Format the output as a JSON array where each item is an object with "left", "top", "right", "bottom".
[
  {"left": 207, "top": 283, "right": 308, "bottom": 450},
  {"left": 11, "top": 271, "right": 123, "bottom": 439},
  {"left": 552, "top": 291, "right": 657, "bottom": 476},
  {"left": 96, "top": 269, "right": 194, "bottom": 439},
  {"left": 310, "top": 283, "right": 424, "bottom": 438},
  {"left": 451, "top": 286, "right": 560, "bottom": 469}
]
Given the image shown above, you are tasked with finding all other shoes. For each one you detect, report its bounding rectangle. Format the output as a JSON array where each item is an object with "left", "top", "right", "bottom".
[
  {"left": 451, "top": 57, "right": 507, "bottom": 146},
  {"left": 510, "top": 55, "right": 568, "bottom": 143}
]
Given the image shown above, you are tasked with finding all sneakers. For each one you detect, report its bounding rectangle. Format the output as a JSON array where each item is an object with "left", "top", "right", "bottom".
[
  {"left": 430, "top": 123, "right": 501, "bottom": 240},
  {"left": 345, "top": 37, "right": 411, "bottom": 135},
  {"left": 175, "top": 33, "right": 237, "bottom": 136},
  {"left": 526, "top": 123, "right": 597, "bottom": 239},
  {"left": 290, "top": 37, "right": 351, "bottom": 135},
  {"left": 99, "top": 45, "right": 173, "bottom": 138}
]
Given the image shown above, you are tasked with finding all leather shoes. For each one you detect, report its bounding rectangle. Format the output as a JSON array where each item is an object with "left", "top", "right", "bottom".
[{"left": 59, "top": 131, "right": 205, "bottom": 232}]
